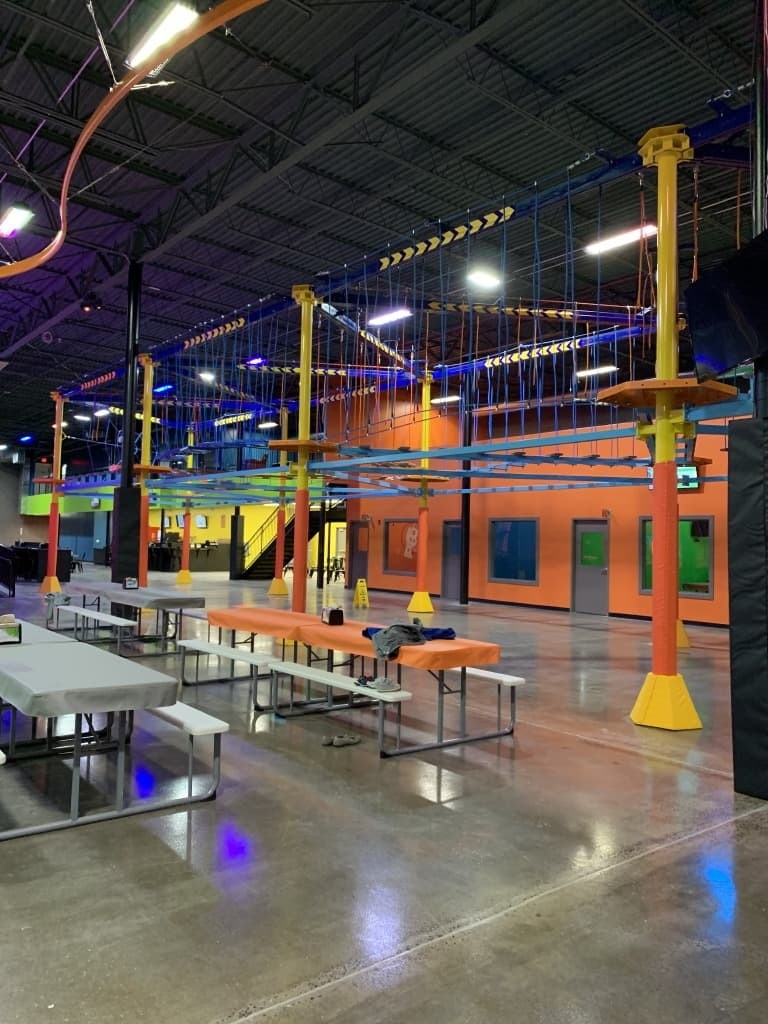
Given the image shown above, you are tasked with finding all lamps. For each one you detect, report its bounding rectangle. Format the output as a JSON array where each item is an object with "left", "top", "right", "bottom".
[
  {"left": 367, "top": 307, "right": 416, "bottom": 328},
  {"left": 584, "top": 222, "right": 660, "bottom": 256},
  {"left": 0, "top": 201, "right": 36, "bottom": 239},
  {"left": 122, "top": 0, "right": 200, "bottom": 79},
  {"left": 0, "top": 295, "right": 286, "bottom": 452}
]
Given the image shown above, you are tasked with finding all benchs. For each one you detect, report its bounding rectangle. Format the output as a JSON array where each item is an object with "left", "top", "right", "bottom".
[
  {"left": 160, "top": 606, "right": 223, "bottom": 645},
  {"left": 45, "top": 603, "right": 140, "bottom": 658},
  {"left": 145, "top": 700, "right": 230, "bottom": 800},
  {"left": 448, "top": 665, "right": 527, "bottom": 732},
  {"left": 178, "top": 638, "right": 414, "bottom": 758}
]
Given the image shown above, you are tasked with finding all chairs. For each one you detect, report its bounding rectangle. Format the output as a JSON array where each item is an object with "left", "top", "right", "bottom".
[
  {"left": 283, "top": 556, "right": 346, "bottom": 585},
  {"left": 72, "top": 552, "right": 87, "bottom": 573}
]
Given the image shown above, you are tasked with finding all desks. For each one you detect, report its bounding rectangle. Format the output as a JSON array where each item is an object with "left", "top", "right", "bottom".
[
  {"left": 0, "top": 615, "right": 177, "bottom": 822},
  {"left": 145, "top": 542, "right": 231, "bottom": 572},
  {"left": 9, "top": 544, "right": 72, "bottom": 583},
  {"left": 64, "top": 582, "right": 206, "bottom": 657},
  {"left": 206, "top": 607, "right": 501, "bottom": 749}
]
